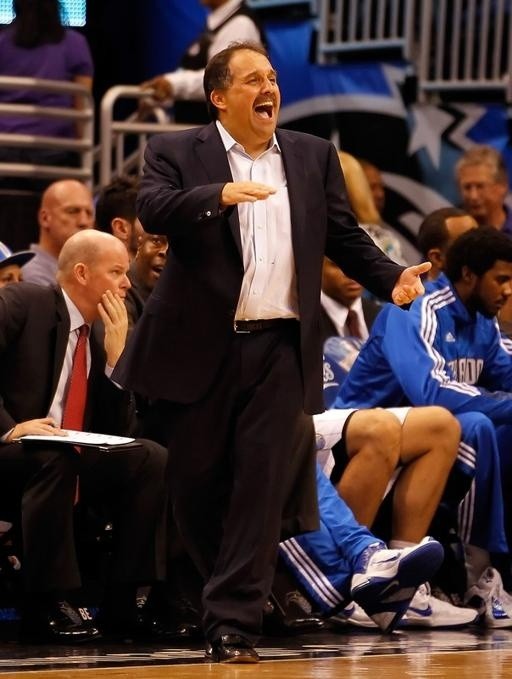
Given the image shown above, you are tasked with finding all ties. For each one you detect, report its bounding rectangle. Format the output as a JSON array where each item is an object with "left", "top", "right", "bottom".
[
  {"left": 346, "top": 310, "right": 361, "bottom": 338},
  {"left": 60, "top": 325, "right": 89, "bottom": 431}
]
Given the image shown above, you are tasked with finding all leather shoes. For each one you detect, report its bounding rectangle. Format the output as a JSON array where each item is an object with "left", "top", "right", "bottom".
[
  {"left": 206, "top": 636, "right": 260, "bottom": 664},
  {"left": 115, "top": 596, "right": 203, "bottom": 644},
  {"left": 18, "top": 600, "right": 102, "bottom": 645}
]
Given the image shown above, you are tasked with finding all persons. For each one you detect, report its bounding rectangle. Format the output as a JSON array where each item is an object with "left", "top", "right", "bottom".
[
  {"left": 277, "top": 148, "right": 511, "bottom": 664},
  {"left": 139, "top": 0, "right": 271, "bottom": 123},
  {"left": 0, "top": 1, "right": 94, "bottom": 253},
  {"left": 0, "top": 179, "right": 203, "bottom": 642},
  {"left": 123, "top": 49, "right": 432, "bottom": 665}
]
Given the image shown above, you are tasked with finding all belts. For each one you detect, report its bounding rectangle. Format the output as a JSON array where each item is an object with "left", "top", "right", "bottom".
[{"left": 231, "top": 321, "right": 297, "bottom": 335}]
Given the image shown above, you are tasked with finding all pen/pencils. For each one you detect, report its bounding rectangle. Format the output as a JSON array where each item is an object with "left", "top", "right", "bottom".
[{"left": 48, "top": 423, "right": 58, "bottom": 429}]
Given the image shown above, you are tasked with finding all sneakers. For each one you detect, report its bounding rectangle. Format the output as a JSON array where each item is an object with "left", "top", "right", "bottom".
[{"left": 329, "top": 536, "right": 512, "bottom": 635}]
